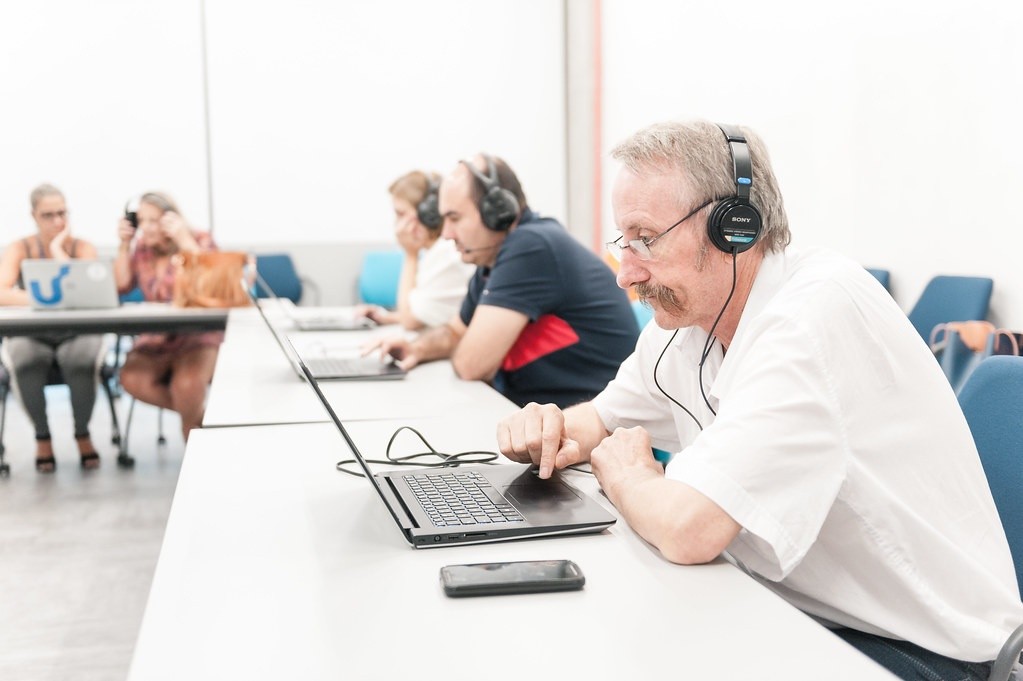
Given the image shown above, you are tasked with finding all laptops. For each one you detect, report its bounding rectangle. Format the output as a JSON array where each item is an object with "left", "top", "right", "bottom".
[
  {"left": 20, "top": 258, "right": 122, "bottom": 310},
  {"left": 241, "top": 264, "right": 617, "bottom": 549}
]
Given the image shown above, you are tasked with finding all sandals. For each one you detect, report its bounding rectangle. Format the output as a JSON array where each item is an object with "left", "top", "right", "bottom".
[
  {"left": 75, "top": 432, "right": 100, "bottom": 470},
  {"left": 34, "top": 433, "right": 57, "bottom": 473}
]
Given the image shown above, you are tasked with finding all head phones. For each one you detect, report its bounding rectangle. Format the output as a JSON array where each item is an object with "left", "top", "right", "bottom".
[
  {"left": 417, "top": 172, "right": 445, "bottom": 229},
  {"left": 123, "top": 193, "right": 139, "bottom": 230},
  {"left": 460, "top": 152, "right": 519, "bottom": 231},
  {"left": 706, "top": 122, "right": 763, "bottom": 255}
]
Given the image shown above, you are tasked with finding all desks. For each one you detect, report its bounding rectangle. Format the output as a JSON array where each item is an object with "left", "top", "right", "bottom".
[
  {"left": 0, "top": 304, "right": 521, "bottom": 428},
  {"left": 128, "top": 431, "right": 907, "bottom": 681}
]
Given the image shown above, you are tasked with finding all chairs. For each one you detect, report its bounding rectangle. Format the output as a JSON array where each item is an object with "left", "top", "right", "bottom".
[
  {"left": 641, "top": 264, "right": 1023, "bottom": 681},
  {"left": 0, "top": 256, "right": 299, "bottom": 473},
  {"left": 356, "top": 249, "right": 403, "bottom": 309}
]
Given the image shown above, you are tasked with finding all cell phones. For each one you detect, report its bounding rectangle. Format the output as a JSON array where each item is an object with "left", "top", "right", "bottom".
[{"left": 440, "top": 560, "right": 585, "bottom": 597}]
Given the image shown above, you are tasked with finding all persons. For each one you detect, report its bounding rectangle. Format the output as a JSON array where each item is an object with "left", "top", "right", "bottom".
[
  {"left": 112, "top": 191, "right": 223, "bottom": 443},
  {"left": 355, "top": 169, "right": 476, "bottom": 333},
  {"left": 0, "top": 184, "right": 103, "bottom": 472},
  {"left": 495, "top": 122, "right": 1023, "bottom": 681},
  {"left": 361, "top": 155, "right": 639, "bottom": 409}
]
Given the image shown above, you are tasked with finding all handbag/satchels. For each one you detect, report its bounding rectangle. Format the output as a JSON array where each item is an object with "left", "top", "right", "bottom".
[
  {"left": 175, "top": 251, "right": 254, "bottom": 307},
  {"left": 929, "top": 322, "right": 1023, "bottom": 394}
]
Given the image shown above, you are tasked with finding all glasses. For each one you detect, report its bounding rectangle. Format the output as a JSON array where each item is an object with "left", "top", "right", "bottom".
[
  {"left": 606, "top": 195, "right": 732, "bottom": 261},
  {"left": 38, "top": 210, "right": 67, "bottom": 219}
]
